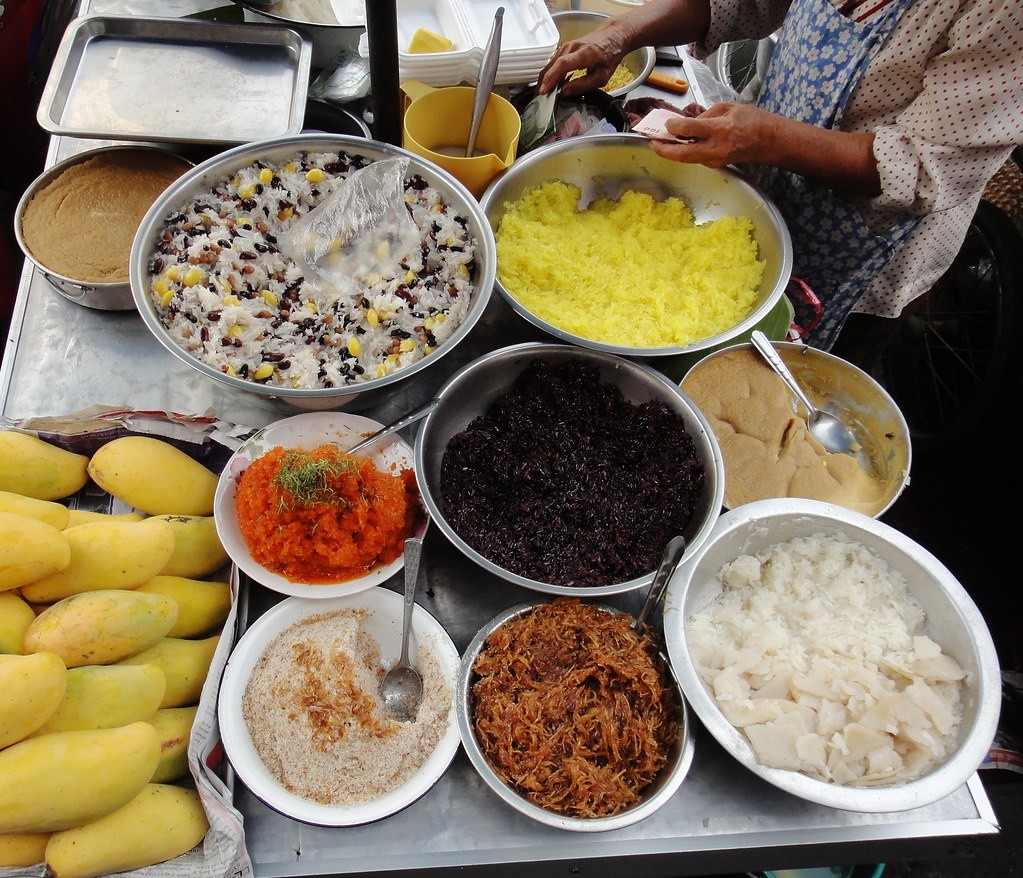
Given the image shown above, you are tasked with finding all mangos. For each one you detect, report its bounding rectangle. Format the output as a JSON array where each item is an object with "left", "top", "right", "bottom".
[{"left": 0, "top": 428, "right": 232, "bottom": 878}]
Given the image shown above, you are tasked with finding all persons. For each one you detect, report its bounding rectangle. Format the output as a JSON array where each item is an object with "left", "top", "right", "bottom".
[{"left": 538, "top": 0, "right": 1023, "bottom": 353}]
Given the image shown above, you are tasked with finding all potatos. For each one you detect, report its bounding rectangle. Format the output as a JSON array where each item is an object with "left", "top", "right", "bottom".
[{"left": 406, "top": 27, "right": 453, "bottom": 53}]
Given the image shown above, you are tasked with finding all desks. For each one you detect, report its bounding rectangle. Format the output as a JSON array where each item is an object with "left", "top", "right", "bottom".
[{"left": 0, "top": 0, "right": 1003, "bottom": 878}]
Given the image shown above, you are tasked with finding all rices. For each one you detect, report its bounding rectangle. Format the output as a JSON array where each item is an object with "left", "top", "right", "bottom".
[
  {"left": 570, "top": 63, "right": 637, "bottom": 92},
  {"left": 440, "top": 358, "right": 703, "bottom": 588},
  {"left": 147, "top": 151, "right": 479, "bottom": 388},
  {"left": 494, "top": 176, "right": 768, "bottom": 347},
  {"left": 685, "top": 517, "right": 927, "bottom": 672}
]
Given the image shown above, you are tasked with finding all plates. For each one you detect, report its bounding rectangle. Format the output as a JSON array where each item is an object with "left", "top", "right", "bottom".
[
  {"left": 217, "top": 587, "right": 462, "bottom": 829},
  {"left": 213, "top": 411, "right": 431, "bottom": 599}
]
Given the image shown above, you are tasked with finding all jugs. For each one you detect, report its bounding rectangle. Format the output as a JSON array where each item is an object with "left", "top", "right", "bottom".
[{"left": 400, "top": 79, "right": 522, "bottom": 205}]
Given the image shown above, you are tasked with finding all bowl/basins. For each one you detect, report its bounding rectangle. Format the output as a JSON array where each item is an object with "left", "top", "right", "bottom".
[
  {"left": 525, "top": 10, "right": 658, "bottom": 95},
  {"left": 129, "top": 133, "right": 498, "bottom": 398},
  {"left": 456, "top": 597, "right": 701, "bottom": 832},
  {"left": 413, "top": 341, "right": 727, "bottom": 597},
  {"left": 301, "top": 97, "right": 373, "bottom": 140},
  {"left": 479, "top": 132, "right": 793, "bottom": 356},
  {"left": 661, "top": 496, "right": 1003, "bottom": 813},
  {"left": 13, "top": 144, "right": 198, "bottom": 309},
  {"left": 679, "top": 339, "right": 912, "bottom": 519}
]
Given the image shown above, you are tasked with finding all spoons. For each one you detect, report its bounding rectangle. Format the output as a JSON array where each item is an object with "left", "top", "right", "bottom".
[
  {"left": 750, "top": 330, "right": 859, "bottom": 457},
  {"left": 381, "top": 539, "right": 423, "bottom": 723}
]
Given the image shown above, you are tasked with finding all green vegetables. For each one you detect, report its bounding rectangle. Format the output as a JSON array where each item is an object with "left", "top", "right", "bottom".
[{"left": 272, "top": 446, "right": 366, "bottom": 535}]
